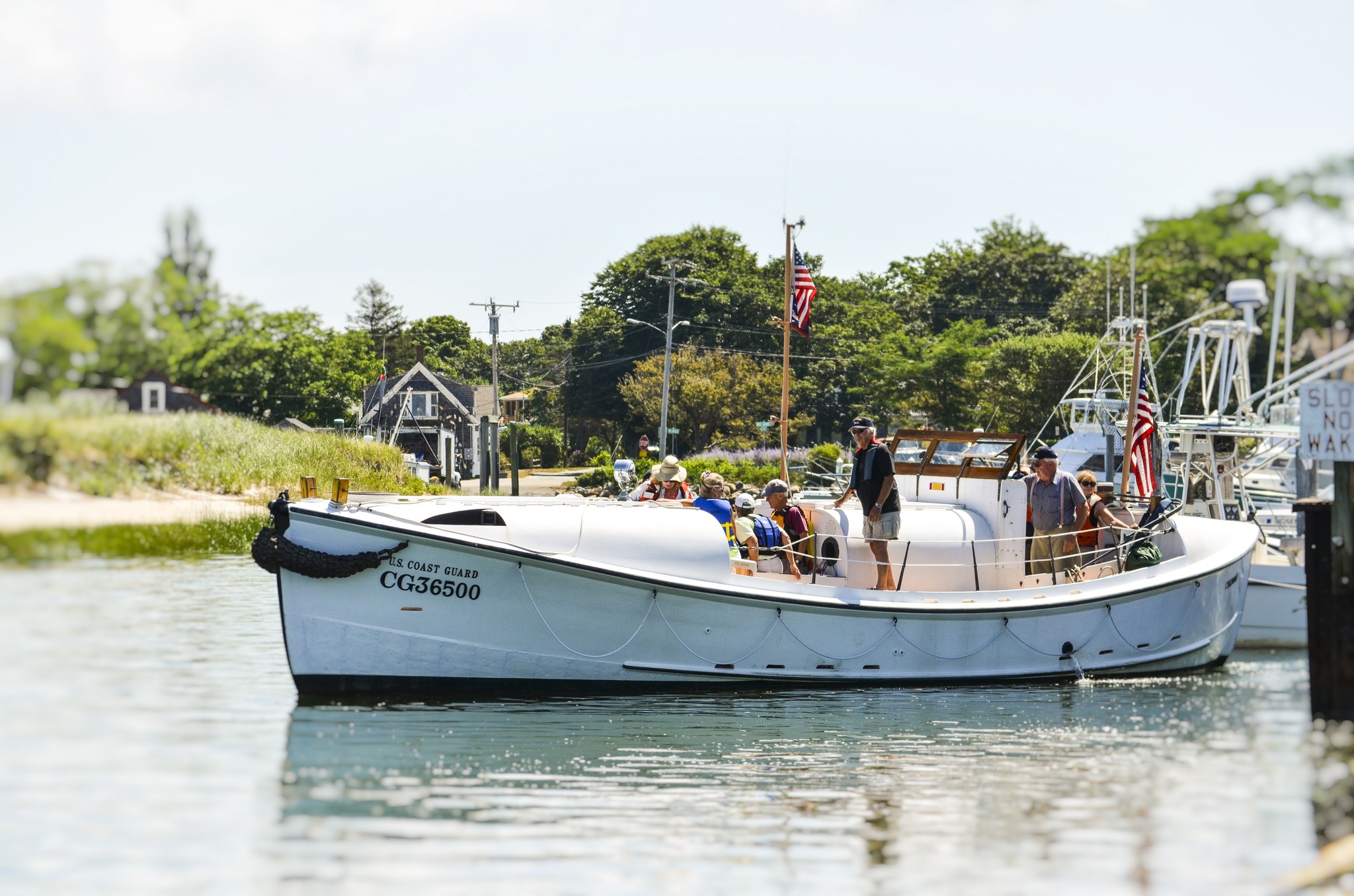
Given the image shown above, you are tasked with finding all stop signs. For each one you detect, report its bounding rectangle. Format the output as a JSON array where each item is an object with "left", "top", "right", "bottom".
[{"left": 639, "top": 436, "right": 649, "bottom": 451}]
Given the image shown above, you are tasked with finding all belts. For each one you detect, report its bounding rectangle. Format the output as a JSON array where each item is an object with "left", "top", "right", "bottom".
[{"left": 758, "top": 556, "right": 778, "bottom": 561}]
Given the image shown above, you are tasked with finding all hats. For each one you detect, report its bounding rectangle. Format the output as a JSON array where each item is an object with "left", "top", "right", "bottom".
[
  {"left": 734, "top": 493, "right": 755, "bottom": 508},
  {"left": 652, "top": 456, "right": 687, "bottom": 482},
  {"left": 758, "top": 479, "right": 788, "bottom": 497},
  {"left": 643, "top": 470, "right": 652, "bottom": 482},
  {"left": 848, "top": 416, "right": 874, "bottom": 432},
  {"left": 1027, "top": 446, "right": 1058, "bottom": 460}
]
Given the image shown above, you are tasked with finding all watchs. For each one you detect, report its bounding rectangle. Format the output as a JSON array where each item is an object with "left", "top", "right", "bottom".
[
  {"left": 875, "top": 503, "right": 883, "bottom": 508},
  {"left": 1072, "top": 534, "right": 1078, "bottom": 536}
]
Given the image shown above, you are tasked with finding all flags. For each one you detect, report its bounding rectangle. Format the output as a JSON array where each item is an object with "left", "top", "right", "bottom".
[
  {"left": 790, "top": 240, "right": 816, "bottom": 341},
  {"left": 1130, "top": 346, "right": 1157, "bottom": 502}
]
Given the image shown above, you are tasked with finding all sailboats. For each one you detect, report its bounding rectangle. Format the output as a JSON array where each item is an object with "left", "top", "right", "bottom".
[{"left": 1000, "top": 238, "right": 1353, "bottom": 651}]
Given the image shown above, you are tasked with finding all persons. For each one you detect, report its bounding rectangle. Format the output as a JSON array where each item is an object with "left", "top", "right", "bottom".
[
  {"left": 832, "top": 417, "right": 901, "bottom": 591},
  {"left": 734, "top": 493, "right": 801, "bottom": 580},
  {"left": 1074, "top": 469, "right": 1139, "bottom": 567},
  {"left": 655, "top": 470, "right": 741, "bottom": 559},
  {"left": 628, "top": 456, "right": 694, "bottom": 502},
  {"left": 1019, "top": 445, "right": 1089, "bottom": 574},
  {"left": 761, "top": 478, "right": 810, "bottom": 575}
]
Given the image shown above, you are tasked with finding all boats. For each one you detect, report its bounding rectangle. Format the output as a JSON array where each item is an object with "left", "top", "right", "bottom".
[{"left": 250, "top": 212, "right": 1260, "bottom": 703}]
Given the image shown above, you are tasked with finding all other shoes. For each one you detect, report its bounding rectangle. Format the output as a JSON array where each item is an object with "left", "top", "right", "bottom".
[{"left": 866, "top": 586, "right": 876, "bottom": 590}]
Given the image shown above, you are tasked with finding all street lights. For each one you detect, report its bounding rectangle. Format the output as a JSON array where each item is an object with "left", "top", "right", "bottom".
[{"left": 625, "top": 317, "right": 691, "bottom": 463}]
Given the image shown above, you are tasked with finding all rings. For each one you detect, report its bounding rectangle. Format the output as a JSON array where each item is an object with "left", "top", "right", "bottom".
[{"left": 874, "top": 517, "right": 876, "bottom": 518}]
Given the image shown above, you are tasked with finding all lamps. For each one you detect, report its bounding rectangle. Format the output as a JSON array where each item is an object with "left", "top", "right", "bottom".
[{"left": 613, "top": 459, "right": 638, "bottom": 502}]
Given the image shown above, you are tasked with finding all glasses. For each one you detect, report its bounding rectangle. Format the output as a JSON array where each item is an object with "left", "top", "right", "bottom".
[
  {"left": 1080, "top": 481, "right": 1096, "bottom": 487},
  {"left": 663, "top": 480, "right": 677, "bottom": 483},
  {"left": 710, "top": 485, "right": 724, "bottom": 494},
  {"left": 1032, "top": 461, "right": 1046, "bottom": 467},
  {"left": 851, "top": 427, "right": 867, "bottom": 434}
]
